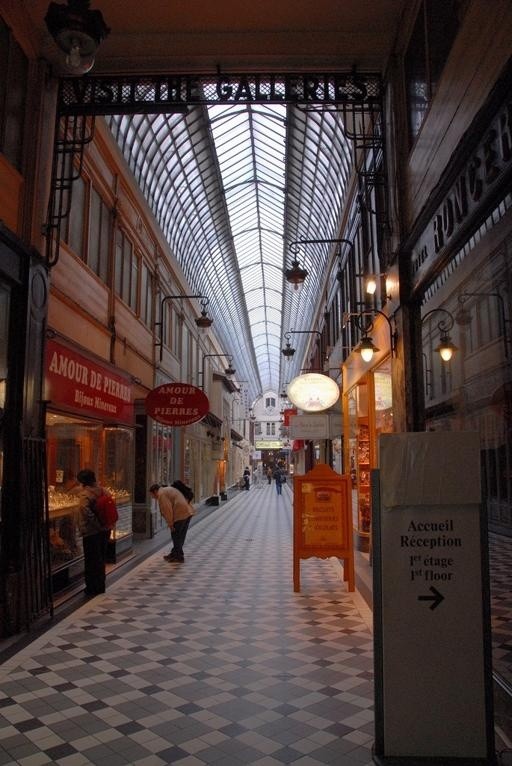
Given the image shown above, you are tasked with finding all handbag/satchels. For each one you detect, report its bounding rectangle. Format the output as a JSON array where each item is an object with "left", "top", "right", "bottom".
[
  {"left": 104, "top": 538, "right": 117, "bottom": 565},
  {"left": 280, "top": 477, "right": 286, "bottom": 483}
]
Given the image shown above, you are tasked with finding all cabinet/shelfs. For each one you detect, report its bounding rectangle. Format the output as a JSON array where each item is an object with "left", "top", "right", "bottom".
[
  {"left": 48, "top": 404, "right": 104, "bottom": 594},
  {"left": 104, "top": 425, "right": 136, "bottom": 563}
]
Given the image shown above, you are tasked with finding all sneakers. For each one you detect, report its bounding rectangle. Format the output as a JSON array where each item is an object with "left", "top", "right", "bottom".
[
  {"left": 164, "top": 553, "right": 174, "bottom": 561},
  {"left": 167, "top": 557, "right": 184, "bottom": 564}
]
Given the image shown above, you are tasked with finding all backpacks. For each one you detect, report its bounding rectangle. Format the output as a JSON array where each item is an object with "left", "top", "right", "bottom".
[
  {"left": 85, "top": 486, "right": 119, "bottom": 529},
  {"left": 170, "top": 479, "right": 194, "bottom": 504}
]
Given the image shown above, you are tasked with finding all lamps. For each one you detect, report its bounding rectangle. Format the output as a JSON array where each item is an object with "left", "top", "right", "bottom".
[
  {"left": 44, "top": 3, "right": 110, "bottom": 82},
  {"left": 150, "top": 285, "right": 217, "bottom": 362},
  {"left": 350, "top": 306, "right": 399, "bottom": 368},
  {"left": 280, "top": 329, "right": 326, "bottom": 369},
  {"left": 195, "top": 351, "right": 238, "bottom": 391},
  {"left": 282, "top": 231, "right": 360, "bottom": 331},
  {"left": 415, "top": 302, "right": 460, "bottom": 364},
  {"left": 231, "top": 396, "right": 258, "bottom": 423},
  {"left": 279, "top": 380, "right": 291, "bottom": 399},
  {"left": 366, "top": 272, "right": 389, "bottom": 294}
]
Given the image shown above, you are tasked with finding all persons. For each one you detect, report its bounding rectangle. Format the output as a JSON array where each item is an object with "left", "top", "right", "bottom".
[
  {"left": 242, "top": 466, "right": 283, "bottom": 495},
  {"left": 151, "top": 484, "right": 196, "bottom": 563},
  {"left": 78, "top": 470, "right": 111, "bottom": 595}
]
